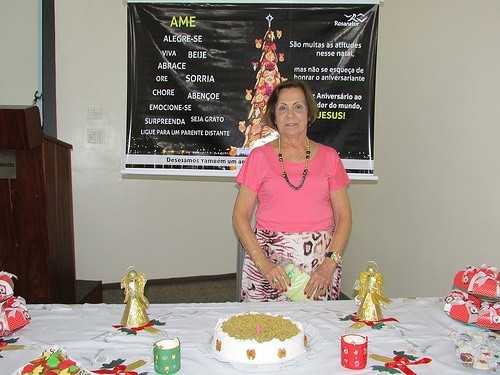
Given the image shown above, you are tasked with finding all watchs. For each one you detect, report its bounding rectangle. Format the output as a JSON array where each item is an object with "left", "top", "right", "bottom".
[{"left": 325, "top": 251, "right": 342, "bottom": 264}]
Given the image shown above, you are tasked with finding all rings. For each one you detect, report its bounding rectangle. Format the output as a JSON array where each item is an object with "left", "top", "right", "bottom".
[{"left": 273, "top": 278, "right": 279, "bottom": 285}]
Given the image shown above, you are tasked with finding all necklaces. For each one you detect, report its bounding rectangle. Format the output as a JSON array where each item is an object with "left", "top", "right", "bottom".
[{"left": 278, "top": 135, "right": 311, "bottom": 190}]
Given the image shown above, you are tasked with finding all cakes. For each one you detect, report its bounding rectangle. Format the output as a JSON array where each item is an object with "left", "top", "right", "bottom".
[{"left": 212, "top": 312, "right": 307, "bottom": 364}]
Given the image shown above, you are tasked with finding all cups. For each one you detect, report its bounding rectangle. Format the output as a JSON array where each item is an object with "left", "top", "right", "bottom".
[
  {"left": 153, "top": 337, "right": 181, "bottom": 374},
  {"left": 339, "top": 334, "right": 368, "bottom": 370}
]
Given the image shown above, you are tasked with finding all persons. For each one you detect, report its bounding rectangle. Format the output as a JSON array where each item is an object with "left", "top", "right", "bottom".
[{"left": 232, "top": 79, "right": 353, "bottom": 302}]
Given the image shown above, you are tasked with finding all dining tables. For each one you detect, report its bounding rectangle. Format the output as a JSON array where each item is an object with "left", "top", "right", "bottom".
[{"left": 0, "top": 297, "right": 500, "bottom": 375}]
